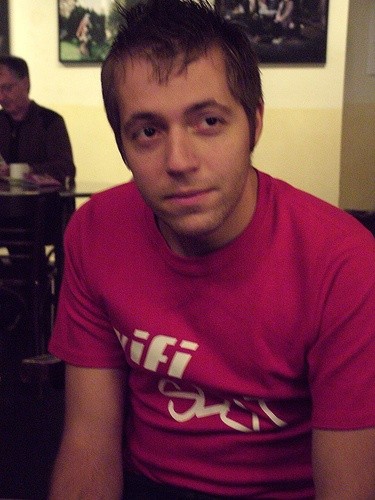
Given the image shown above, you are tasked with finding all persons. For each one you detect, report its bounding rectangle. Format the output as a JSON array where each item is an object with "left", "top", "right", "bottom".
[
  {"left": 1, "top": 55, "right": 76, "bottom": 383},
  {"left": 48, "top": 0, "right": 374, "bottom": 500}
]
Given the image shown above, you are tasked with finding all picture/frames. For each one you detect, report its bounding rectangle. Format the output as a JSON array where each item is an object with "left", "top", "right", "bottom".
[
  {"left": 214, "top": 0, "right": 330, "bottom": 64},
  {"left": 57, "top": 0, "right": 127, "bottom": 62}
]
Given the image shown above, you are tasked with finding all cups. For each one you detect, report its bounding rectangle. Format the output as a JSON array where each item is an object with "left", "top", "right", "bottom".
[{"left": 8, "top": 162, "right": 30, "bottom": 180}]
